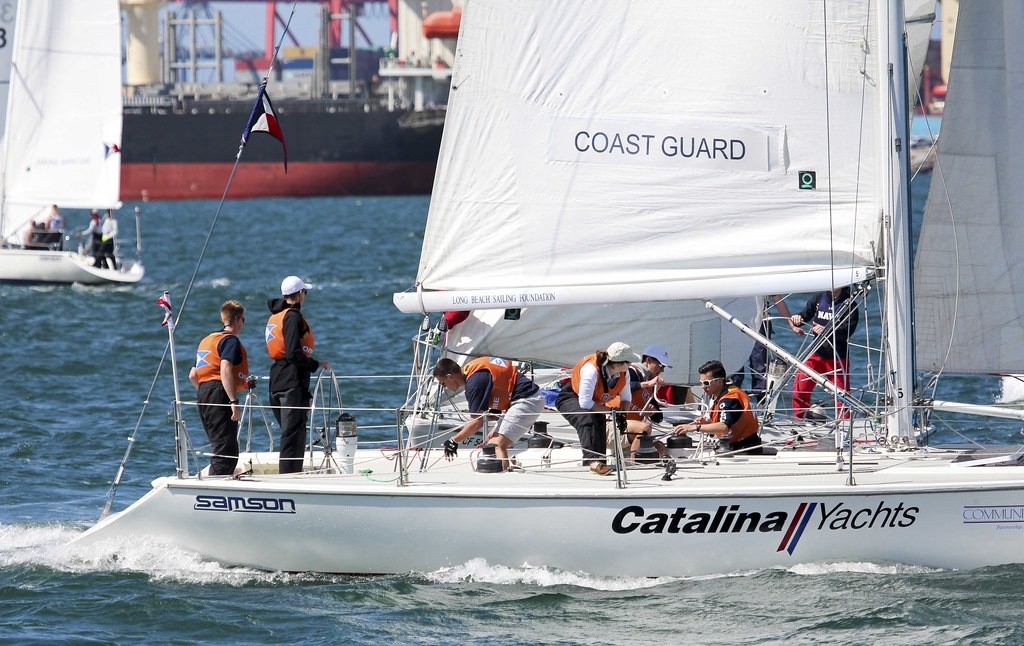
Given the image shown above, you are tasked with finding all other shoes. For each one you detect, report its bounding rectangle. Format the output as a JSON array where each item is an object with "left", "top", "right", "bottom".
[
  {"left": 660, "top": 453, "right": 676, "bottom": 467},
  {"left": 590, "top": 462, "right": 613, "bottom": 476}
]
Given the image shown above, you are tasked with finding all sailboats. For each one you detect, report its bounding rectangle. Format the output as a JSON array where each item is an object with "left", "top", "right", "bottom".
[
  {"left": 0, "top": 0, "right": 148, "bottom": 284},
  {"left": 66, "top": 0, "right": 1024, "bottom": 581},
  {"left": 899, "top": 0, "right": 941, "bottom": 174}
]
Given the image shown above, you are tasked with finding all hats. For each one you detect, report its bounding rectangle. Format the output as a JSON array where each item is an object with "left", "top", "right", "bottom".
[
  {"left": 602, "top": 342, "right": 642, "bottom": 366},
  {"left": 642, "top": 344, "right": 673, "bottom": 368},
  {"left": 281, "top": 276, "right": 312, "bottom": 296}
]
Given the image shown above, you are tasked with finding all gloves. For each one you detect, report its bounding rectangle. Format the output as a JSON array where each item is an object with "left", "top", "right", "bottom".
[{"left": 441, "top": 438, "right": 458, "bottom": 462}]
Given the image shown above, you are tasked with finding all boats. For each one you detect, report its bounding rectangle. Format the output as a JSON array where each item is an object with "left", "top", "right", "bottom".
[{"left": 119, "top": 1, "right": 458, "bottom": 200}]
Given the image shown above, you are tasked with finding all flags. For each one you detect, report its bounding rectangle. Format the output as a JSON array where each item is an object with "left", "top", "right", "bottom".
[{"left": 236, "top": 82, "right": 287, "bottom": 173}]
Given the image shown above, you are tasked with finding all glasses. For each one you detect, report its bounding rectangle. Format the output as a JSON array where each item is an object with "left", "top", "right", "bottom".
[
  {"left": 302, "top": 290, "right": 307, "bottom": 295},
  {"left": 440, "top": 377, "right": 445, "bottom": 386},
  {"left": 699, "top": 377, "right": 725, "bottom": 386},
  {"left": 240, "top": 316, "right": 245, "bottom": 322},
  {"left": 652, "top": 360, "right": 665, "bottom": 369}
]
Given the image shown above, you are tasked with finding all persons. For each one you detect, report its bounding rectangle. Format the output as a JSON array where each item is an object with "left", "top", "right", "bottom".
[
  {"left": 555, "top": 341, "right": 641, "bottom": 476},
  {"left": 189, "top": 300, "right": 249, "bottom": 476},
  {"left": 74, "top": 210, "right": 118, "bottom": 269},
  {"left": 433, "top": 357, "right": 545, "bottom": 470},
  {"left": 732, "top": 295, "right": 805, "bottom": 424},
  {"left": 606, "top": 345, "right": 673, "bottom": 464},
  {"left": 674, "top": 360, "right": 763, "bottom": 455},
  {"left": 22, "top": 221, "right": 36, "bottom": 249},
  {"left": 787, "top": 286, "right": 859, "bottom": 446},
  {"left": 44, "top": 204, "right": 65, "bottom": 251},
  {"left": 430, "top": 286, "right": 471, "bottom": 344},
  {"left": 266, "top": 276, "right": 321, "bottom": 473}
]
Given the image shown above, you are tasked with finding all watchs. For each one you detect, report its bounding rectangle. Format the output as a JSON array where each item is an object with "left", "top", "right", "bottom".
[
  {"left": 696, "top": 424, "right": 701, "bottom": 432},
  {"left": 229, "top": 399, "right": 239, "bottom": 404}
]
